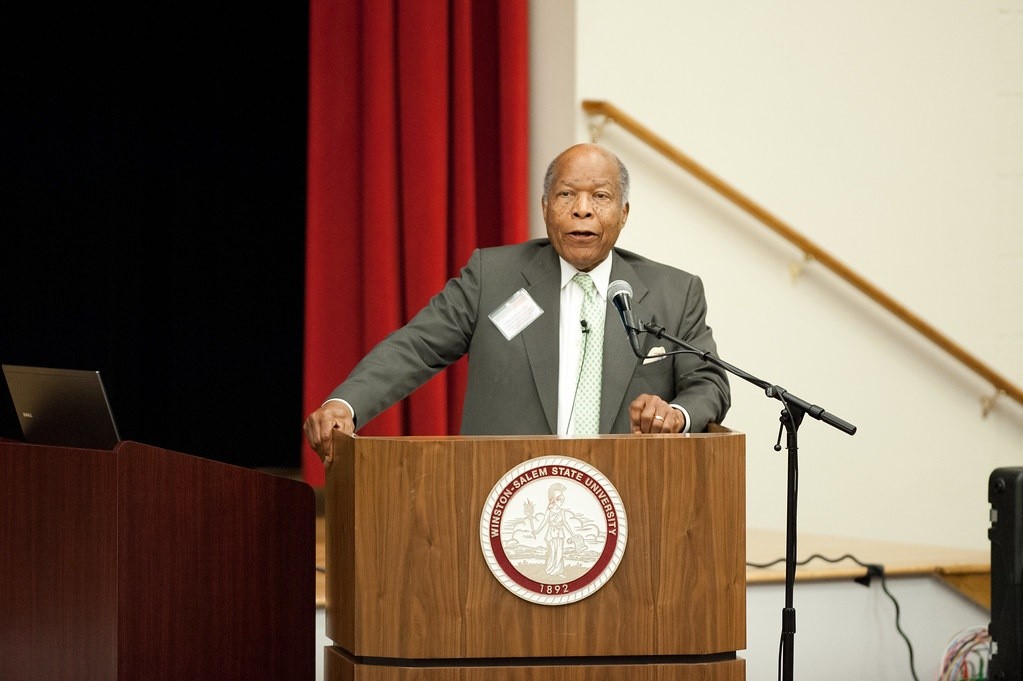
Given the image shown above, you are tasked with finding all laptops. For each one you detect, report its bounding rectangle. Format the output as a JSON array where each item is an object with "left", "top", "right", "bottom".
[{"left": 2, "top": 366, "right": 122, "bottom": 449}]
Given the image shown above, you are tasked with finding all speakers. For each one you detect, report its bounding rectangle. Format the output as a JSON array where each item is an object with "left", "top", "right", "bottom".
[{"left": 988, "top": 465, "right": 1023, "bottom": 681}]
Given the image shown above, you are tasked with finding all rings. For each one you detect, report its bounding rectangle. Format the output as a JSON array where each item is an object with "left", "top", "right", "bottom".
[{"left": 654, "top": 416, "right": 664, "bottom": 422}]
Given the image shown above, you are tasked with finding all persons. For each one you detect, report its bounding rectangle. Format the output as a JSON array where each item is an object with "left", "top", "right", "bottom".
[{"left": 303, "top": 142, "right": 731, "bottom": 464}]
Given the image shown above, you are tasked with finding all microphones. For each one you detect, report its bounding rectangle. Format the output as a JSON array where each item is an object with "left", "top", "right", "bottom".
[
  {"left": 607, "top": 280, "right": 646, "bottom": 359},
  {"left": 581, "top": 319, "right": 589, "bottom": 334}
]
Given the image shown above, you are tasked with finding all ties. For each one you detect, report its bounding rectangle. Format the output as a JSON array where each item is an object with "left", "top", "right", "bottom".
[{"left": 565, "top": 274, "right": 605, "bottom": 438}]
